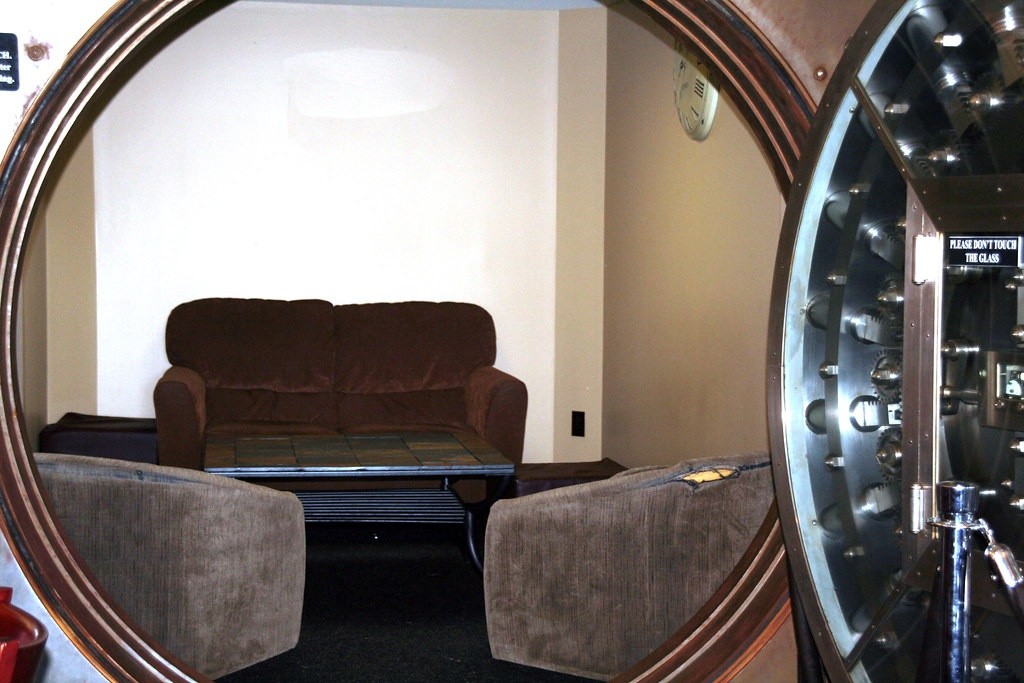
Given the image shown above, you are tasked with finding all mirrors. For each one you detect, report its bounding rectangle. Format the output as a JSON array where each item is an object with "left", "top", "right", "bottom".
[{"left": 0, "top": 0, "right": 826, "bottom": 683}]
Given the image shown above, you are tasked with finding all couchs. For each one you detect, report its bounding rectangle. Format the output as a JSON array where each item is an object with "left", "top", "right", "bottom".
[
  {"left": 483, "top": 447, "right": 784, "bottom": 679},
  {"left": 29, "top": 453, "right": 306, "bottom": 680},
  {"left": 152, "top": 298, "right": 530, "bottom": 574}
]
